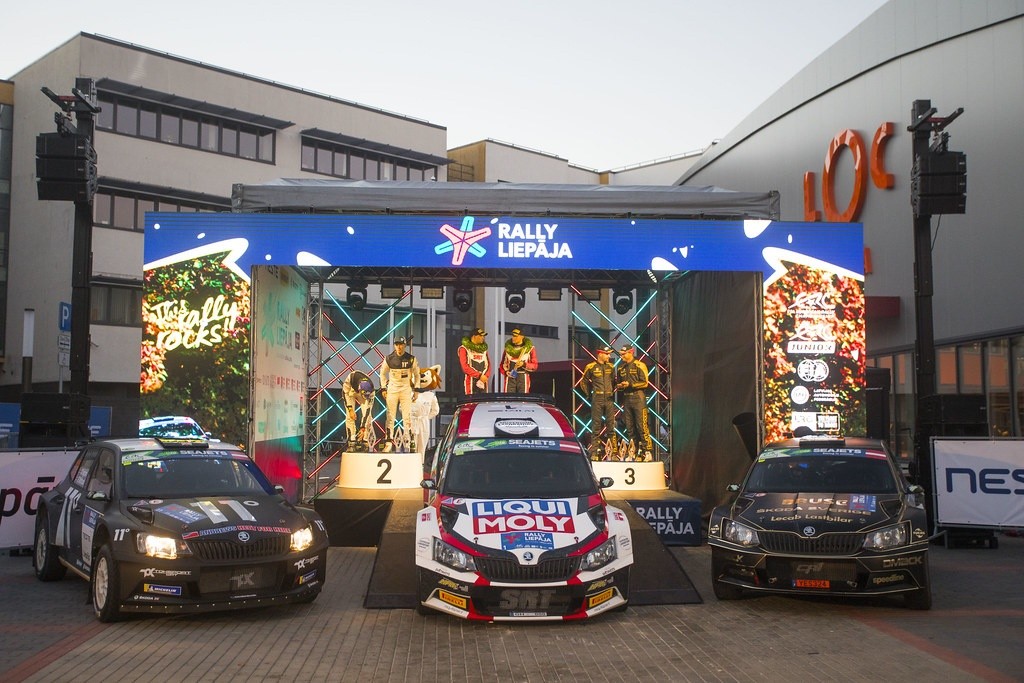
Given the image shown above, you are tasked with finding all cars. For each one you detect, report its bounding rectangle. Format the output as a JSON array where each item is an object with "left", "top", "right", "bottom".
[
  {"left": 33, "top": 436, "right": 329, "bottom": 623},
  {"left": 414, "top": 394, "right": 635, "bottom": 623},
  {"left": 138, "top": 414, "right": 222, "bottom": 442},
  {"left": 707, "top": 435, "right": 932, "bottom": 610}
]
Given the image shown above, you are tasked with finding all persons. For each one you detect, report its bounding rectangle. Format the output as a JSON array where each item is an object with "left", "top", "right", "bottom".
[
  {"left": 498, "top": 329, "right": 538, "bottom": 393},
  {"left": 341, "top": 370, "right": 375, "bottom": 453},
  {"left": 457, "top": 328, "right": 492, "bottom": 394},
  {"left": 580, "top": 345, "right": 618, "bottom": 461},
  {"left": 617, "top": 344, "right": 653, "bottom": 462},
  {"left": 380, "top": 335, "right": 420, "bottom": 453}
]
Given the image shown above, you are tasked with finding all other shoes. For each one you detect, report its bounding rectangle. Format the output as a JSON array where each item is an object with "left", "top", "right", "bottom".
[
  {"left": 382, "top": 443, "right": 392, "bottom": 453},
  {"left": 611, "top": 454, "right": 620, "bottom": 460},
  {"left": 347, "top": 448, "right": 355, "bottom": 452},
  {"left": 591, "top": 452, "right": 599, "bottom": 461},
  {"left": 626, "top": 453, "right": 634, "bottom": 461},
  {"left": 365, "top": 447, "right": 377, "bottom": 453},
  {"left": 643, "top": 451, "right": 652, "bottom": 462}
]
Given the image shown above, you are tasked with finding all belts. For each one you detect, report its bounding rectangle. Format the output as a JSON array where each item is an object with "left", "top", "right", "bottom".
[{"left": 596, "top": 391, "right": 611, "bottom": 394}]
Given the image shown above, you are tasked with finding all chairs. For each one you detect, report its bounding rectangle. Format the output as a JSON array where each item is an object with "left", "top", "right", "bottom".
[{"left": 779, "top": 464, "right": 885, "bottom": 494}]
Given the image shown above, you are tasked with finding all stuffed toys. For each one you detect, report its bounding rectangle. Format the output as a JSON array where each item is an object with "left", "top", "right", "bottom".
[{"left": 398, "top": 364, "right": 442, "bottom": 468}]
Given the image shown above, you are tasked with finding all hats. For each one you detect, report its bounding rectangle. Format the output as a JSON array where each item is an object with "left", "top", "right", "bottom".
[
  {"left": 394, "top": 336, "right": 406, "bottom": 344},
  {"left": 597, "top": 345, "right": 614, "bottom": 354},
  {"left": 472, "top": 328, "right": 488, "bottom": 336},
  {"left": 620, "top": 344, "right": 634, "bottom": 354},
  {"left": 510, "top": 328, "right": 525, "bottom": 336},
  {"left": 359, "top": 383, "right": 371, "bottom": 400}
]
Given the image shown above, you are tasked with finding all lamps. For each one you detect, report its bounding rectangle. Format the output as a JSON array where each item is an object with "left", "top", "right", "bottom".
[
  {"left": 419, "top": 282, "right": 445, "bottom": 300},
  {"left": 379, "top": 281, "right": 406, "bottom": 300},
  {"left": 504, "top": 285, "right": 527, "bottom": 314},
  {"left": 612, "top": 288, "right": 633, "bottom": 315},
  {"left": 577, "top": 288, "right": 602, "bottom": 301},
  {"left": 537, "top": 287, "right": 563, "bottom": 302},
  {"left": 452, "top": 285, "right": 474, "bottom": 313},
  {"left": 346, "top": 282, "right": 368, "bottom": 312},
  {"left": 635, "top": 269, "right": 658, "bottom": 290}
]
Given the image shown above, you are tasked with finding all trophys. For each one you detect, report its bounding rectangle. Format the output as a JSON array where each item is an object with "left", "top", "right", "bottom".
[{"left": 510, "top": 347, "right": 527, "bottom": 378}]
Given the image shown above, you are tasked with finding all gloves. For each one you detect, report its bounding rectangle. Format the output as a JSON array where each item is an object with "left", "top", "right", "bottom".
[{"left": 476, "top": 381, "right": 484, "bottom": 389}]
